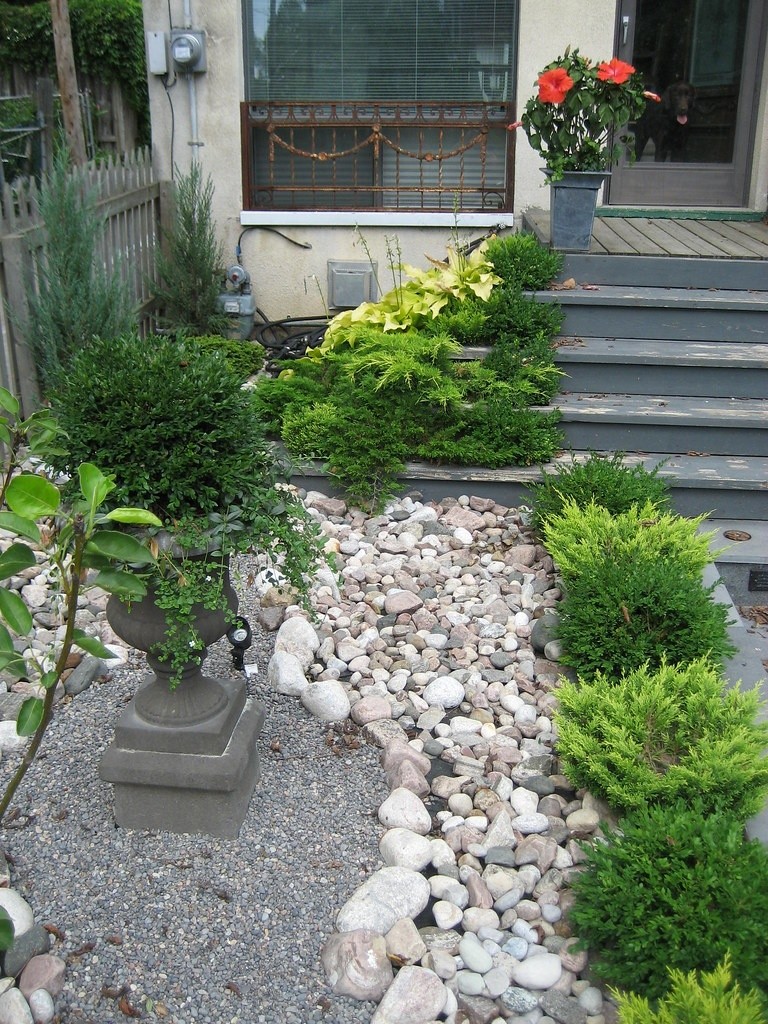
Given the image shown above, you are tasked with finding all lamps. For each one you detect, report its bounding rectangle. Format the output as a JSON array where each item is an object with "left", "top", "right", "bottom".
[{"left": 226, "top": 616, "right": 252, "bottom": 667}]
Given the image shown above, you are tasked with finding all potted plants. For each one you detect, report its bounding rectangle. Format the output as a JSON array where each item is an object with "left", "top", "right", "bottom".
[{"left": 30, "top": 334, "right": 324, "bottom": 836}]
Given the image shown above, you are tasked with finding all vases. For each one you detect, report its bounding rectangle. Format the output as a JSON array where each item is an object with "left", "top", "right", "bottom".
[{"left": 539, "top": 166, "right": 612, "bottom": 252}]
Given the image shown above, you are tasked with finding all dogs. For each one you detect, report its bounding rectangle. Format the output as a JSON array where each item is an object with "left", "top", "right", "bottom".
[{"left": 632, "top": 82, "right": 696, "bottom": 162}]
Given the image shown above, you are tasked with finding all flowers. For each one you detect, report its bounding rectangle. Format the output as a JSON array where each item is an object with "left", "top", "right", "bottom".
[{"left": 522, "top": 48, "right": 660, "bottom": 187}]
